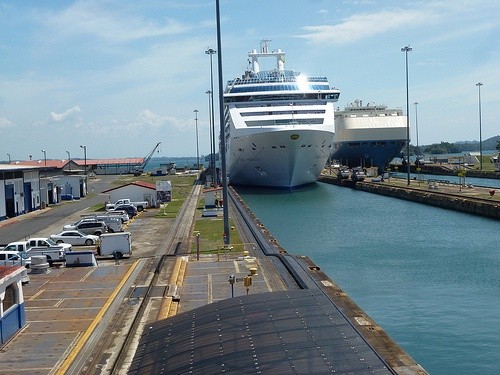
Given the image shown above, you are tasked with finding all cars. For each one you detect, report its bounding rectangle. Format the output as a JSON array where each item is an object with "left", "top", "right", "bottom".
[
  {"left": 49, "top": 230, "right": 99, "bottom": 246},
  {"left": 0, "top": 237, "right": 72, "bottom": 270}
]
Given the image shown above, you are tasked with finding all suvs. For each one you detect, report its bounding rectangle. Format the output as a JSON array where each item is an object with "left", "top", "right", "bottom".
[
  {"left": 70, "top": 204, "right": 138, "bottom": 233},
  {"left": 62, "top": 218, "right": 108, "bottom": 236}
]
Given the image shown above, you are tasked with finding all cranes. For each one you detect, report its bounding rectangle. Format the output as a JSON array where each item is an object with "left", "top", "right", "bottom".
[{"left": 130, "top": 141, "right": 162, "bottom": 177}]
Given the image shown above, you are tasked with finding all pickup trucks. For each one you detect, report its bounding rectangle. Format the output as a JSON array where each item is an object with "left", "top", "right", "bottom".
[{"left": 106, "top": 198, "right": 149, "bottom": 213}]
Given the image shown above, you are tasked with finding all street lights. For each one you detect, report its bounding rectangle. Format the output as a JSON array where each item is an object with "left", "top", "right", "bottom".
[
  {"left": 6, "top": 153, "right": 11, "bottom": 164},
  {"left": 413, "top": 102, "right": 420, "bottom": 181},
  {"left": 204, "top": 48, "right": 218, "bottom": 189},
  {"left": 193, "top": 109, "right": 200, "bottom": 170},
  {"left": 79, "top": 145, "right": 88, "bottom": 195},
  {"left": 65, "top": 150, "right": 71, "bottom": 170},
  {"left": 475, "top": 81, "right": 484, "bottom": 171},
  {"left": 400, "top": 46, "right": 413, "bottom": 187},
  {"left": 206, "top": 90, "right": 214, "bottom": 185},
  {"left": 41, "top": 150, "right": 47, "bottom": 166}
]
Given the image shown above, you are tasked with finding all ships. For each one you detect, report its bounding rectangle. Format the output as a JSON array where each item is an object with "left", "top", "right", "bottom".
[
  {"left": 325, "top": 99, "right": 412, "bottom": 174},
  {"left": 217, "top": 39, "right": 341, "bottom": 192}
]
